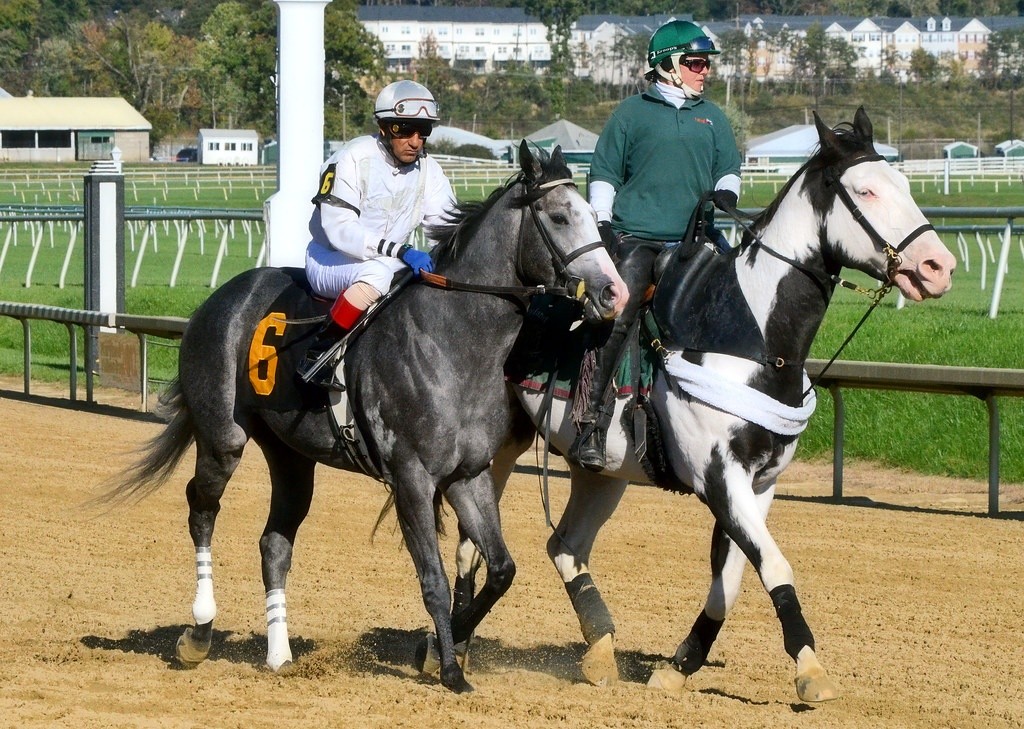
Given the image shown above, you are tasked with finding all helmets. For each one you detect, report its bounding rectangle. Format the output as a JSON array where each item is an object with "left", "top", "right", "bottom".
[
  {"left": 648, "top": 20, "right": 721, "bottom": 68},
  {"left": 374, "top": 79, "right": 440, "bottom": 120}
]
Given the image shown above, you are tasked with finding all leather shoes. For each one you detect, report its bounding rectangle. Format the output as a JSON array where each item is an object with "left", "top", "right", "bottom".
[
  {"left": 296, "top": 354, "right": 346, "bottom": 392},
  {"left": 567, "top": 422, "right": 606, "bottom": 470}
]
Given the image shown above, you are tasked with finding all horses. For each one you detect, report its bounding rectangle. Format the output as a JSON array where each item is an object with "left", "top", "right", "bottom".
[
  {"left": 74, "top": 139, "right": 629, "bottom": 696},
  {"left": 453, "top": 104, "right": 957, "bottom": 703}
]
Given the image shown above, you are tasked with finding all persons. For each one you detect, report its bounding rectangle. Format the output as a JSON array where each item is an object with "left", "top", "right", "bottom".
[
  {"left": 566, "top": 20, "right": 740, "bottom": 474},
  {"left": 295, "top": 80, "right": 461, "bottom": 391}
]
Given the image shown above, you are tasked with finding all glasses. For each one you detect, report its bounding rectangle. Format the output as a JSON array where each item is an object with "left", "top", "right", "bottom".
[
  {"left": 677, "top": 37, "right": 716, "bottom": 51},
  {"left": 681, "top": 59, "right": 710, "bottom": 73},
  {"left": 384, "top": 122, "right": 433, "bottom": 138}
]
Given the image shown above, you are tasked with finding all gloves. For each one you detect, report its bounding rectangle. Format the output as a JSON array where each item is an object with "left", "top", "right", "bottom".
[
  {"left": 397, "top": 245, "right": 435, "bottom": 275},
  {"left": 713, "top": 189, "right": 738, "bottom": 210},
  {"left": 597, "top": 220, "right": 619, "bottom": 256}
]
